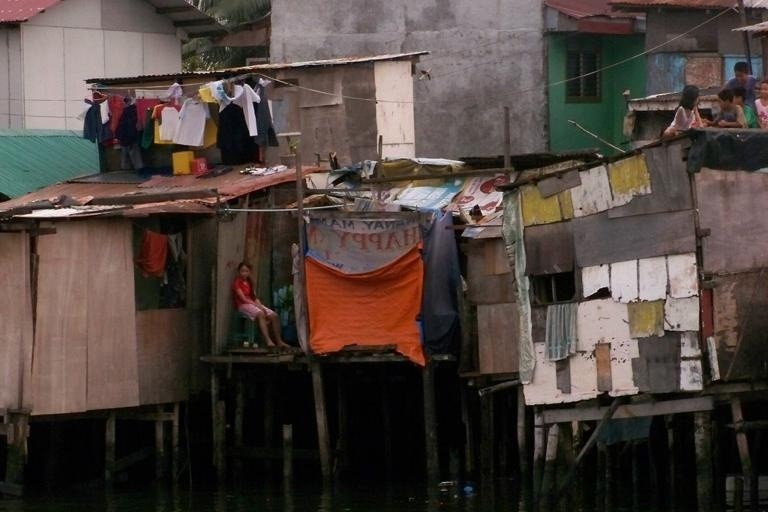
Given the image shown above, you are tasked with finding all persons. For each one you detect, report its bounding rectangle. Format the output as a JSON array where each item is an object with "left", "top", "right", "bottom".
[
  {"left": 702, "top": 88, "right": 750, "bottom": 129},
  {"left": 231, "top": 261, "right": 291, "bottom": 349},
  {"left": 717, "top": 61, "right": 761, "bottom": 107},
  {"left": 663, "top": 83, "right": 705, "bottom": 139},
  {"left": 729, "top": 85, "right": 762, "bottom": 128},
  {"left": 752, "top": 79, "right": 768, "bottom": 130}
]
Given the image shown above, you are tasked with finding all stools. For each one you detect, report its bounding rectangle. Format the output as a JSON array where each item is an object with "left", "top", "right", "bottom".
[{"left": 227, "top": 312, "right": 256, "bottom": 349}]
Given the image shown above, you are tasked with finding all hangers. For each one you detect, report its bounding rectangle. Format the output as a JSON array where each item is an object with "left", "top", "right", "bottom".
[{"left": 90, "top": 86, "right": 107, "bottom": 101}]
